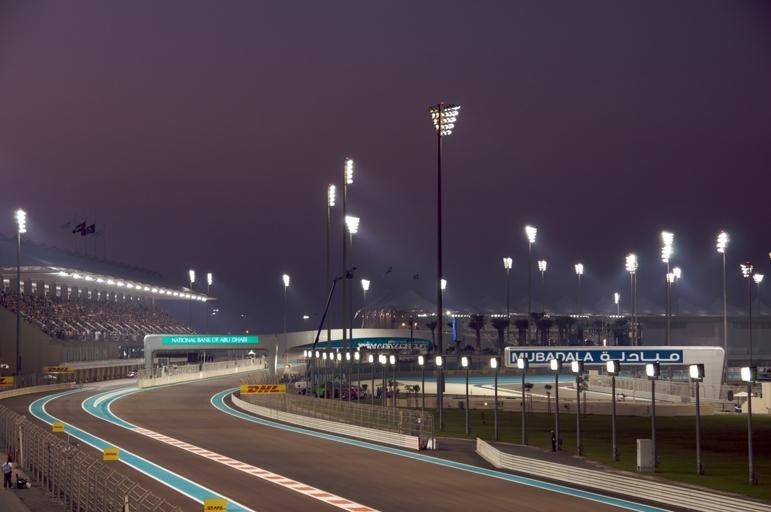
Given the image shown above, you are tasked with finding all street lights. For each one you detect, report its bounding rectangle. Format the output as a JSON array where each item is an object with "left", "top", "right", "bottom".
[
  {"left": 423, "top": 97, "right": 465, "bottom": 391},
  {"left": 13, "top": 206, "right": 28, "bottom": 377},
  {"left": 741, "top": 258, "right": 754, "bottom": 359},
  {"left": 360, "top": 280, "right": 372, "bottom": 322},
  {"left": 188, "top": 269, "right": 196, "bottom": 333},
  {"left": 660, "top": 230, "right": 682, "bottom": 350},
  {"left": 716, "top": 230, "right": 731, "bottom": 365},
  {"left": 303, "top": 349, "right": 398, "bottom": 412},
  {"left": 345, "top": 214, "right": 362, "bottom": 343},
  {"left": 324, "top": 183, "right": 338, "bottom": 342},
  {"left": 204, "top": 272, "right": 212, "bottom": 335},
  {"left": 502, "top": 226, "right": 640, "bottom": 350},
  {"left": 751, "top": 272, "right": 766, "bottom": 358},
  {"left": 342, "top": 154, "right": 355, "bottom": 347},
  {"left": 441, "top": 279, "right": 448, "bottom": 335},
  {"left": 281, "top": 273, "right": 294, "bottom": 363}
]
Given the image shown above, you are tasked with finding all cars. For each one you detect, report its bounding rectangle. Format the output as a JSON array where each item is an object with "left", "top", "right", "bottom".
[{"left": 301, "top": 381, "right": 368, "bottom": 400}]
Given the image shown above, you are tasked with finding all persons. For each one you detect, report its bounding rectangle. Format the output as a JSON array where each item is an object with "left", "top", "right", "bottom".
[
  {"left": 1, "top": 462, "right": 13, "bottom": 488},
  {"left": 549, "top": 429, "right": 556, "bottom": 452},
  {"left": 7, "top": 454, "right": 13, "bottom": 463}
]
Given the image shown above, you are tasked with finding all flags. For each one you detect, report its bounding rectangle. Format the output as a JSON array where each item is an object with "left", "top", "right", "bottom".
[
  {"left": 81, "top": 224, "right": 95, "bottom": 236},
  {"left": 72, "top": 223, "right": 85, "bottom": 233}
]
